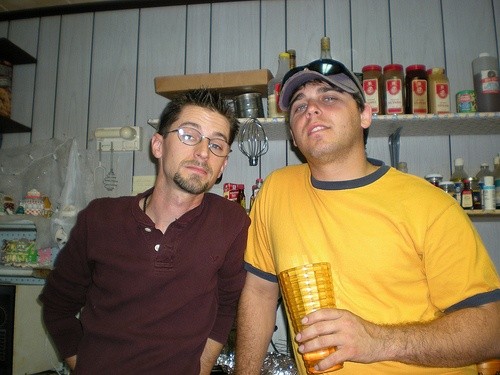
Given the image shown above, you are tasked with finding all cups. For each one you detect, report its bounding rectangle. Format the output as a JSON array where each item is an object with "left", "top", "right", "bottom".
[
  {"left": 227, "top": 92, "right": 265, "bottom": 118},
  {"left": 276, "top": 261, "right": 344, "bottom": 375}
]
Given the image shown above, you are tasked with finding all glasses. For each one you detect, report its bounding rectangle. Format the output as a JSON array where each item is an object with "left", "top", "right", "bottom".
[
  {"left": 281, "top": 59, "right": 365, "bottom": 103},
  {"left": 161, "top": 127, "right": 231, "bottom": 157}
]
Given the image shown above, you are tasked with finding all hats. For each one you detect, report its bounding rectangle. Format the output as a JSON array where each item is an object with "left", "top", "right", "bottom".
[{"left": 278, "top": 58, "right": 366, "bottom": 112}]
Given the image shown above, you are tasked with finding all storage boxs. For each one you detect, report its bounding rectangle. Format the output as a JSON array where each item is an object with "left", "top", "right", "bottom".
[{"left": 154, "top": 68, "right": 275, "bottom": 101}]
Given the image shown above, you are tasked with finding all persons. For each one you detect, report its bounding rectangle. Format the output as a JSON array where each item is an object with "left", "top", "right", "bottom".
[
  {"left": 233, "top": 57, "right": 500, "bottom": 375},
  {"left": 38, "top": 87, "right": 251, "bottom": 375}
]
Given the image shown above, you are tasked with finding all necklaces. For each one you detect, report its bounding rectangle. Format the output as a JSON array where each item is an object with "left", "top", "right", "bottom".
[{"left": 143, "top": 194, "right": 150, "bottom": 212}]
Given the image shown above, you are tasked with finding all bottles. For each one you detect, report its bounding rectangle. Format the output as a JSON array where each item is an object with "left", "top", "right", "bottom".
[
  {"left": 320, "top": 37, "right": 332, "bottom": 60},
  {"left": 472, "top": 53, "right": 500, "bottom": 113},
  {"left": 267, "top": 50, "right": 296, "bottom": 118},
  {"left": 237, "top": 178, "right": 263, "bottom": 212},
  {"left": 397, "top": 156, "right": 500, "bottom": 210}
]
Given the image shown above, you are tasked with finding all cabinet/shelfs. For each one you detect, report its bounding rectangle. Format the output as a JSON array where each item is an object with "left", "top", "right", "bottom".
[{"left": 147, "top": 111, "right": 500, "bottom": 216}]
